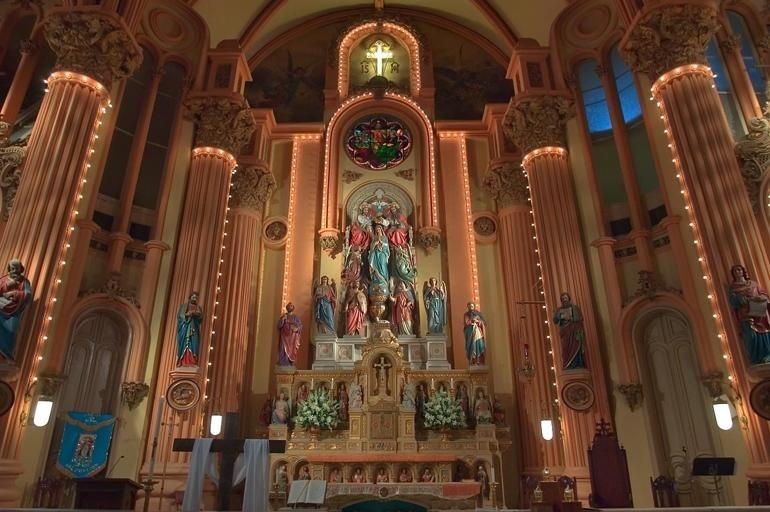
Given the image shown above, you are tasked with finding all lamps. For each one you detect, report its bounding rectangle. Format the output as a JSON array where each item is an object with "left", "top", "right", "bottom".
[
  {"left": 205, "top": 398, "right": 223, "bottom": 435},
  {"left": 515, "top": 298, "right": 546, "bottom": 386},
  {"left": 541, "top": 401, "right": 561, "bottom": 440},
  {"left": 25, "top": 375, "right": 64, "bottom": 428},
  {"left": 711, "top": 378, "right": 746, "bottom": 431}
]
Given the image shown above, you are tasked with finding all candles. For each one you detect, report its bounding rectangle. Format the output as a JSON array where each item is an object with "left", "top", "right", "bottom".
[
  {"left": 451, "top": 377, "right": 453, "bottom": 390},
  {"left": 331, "top": 378, "right": 333, "bottom": 389},
  {"left": 310, "top": 378, "right": 314, "bottom": 389},
  {"left": 432, "top": 378, "right": 435, "bottom": 390},
  {"left": 491, "top": 467, "right": 494, "bottom": 481},
  {"left": 148, "top": 395, "right": 166, "bottom": 480}
]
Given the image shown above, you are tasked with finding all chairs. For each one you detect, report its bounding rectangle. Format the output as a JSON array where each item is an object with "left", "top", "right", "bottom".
[
  {"left": 519, "top": 475, "right": 538, "bottom": 511},
  {"left": 650, "top": 475, "right": 681, "bottom": 507},
  {"left": 747, "top": 481, "right": 770, "bottom": 505},
  {"left": 554, "top": 475, "right": 580, "bottom": 512}
]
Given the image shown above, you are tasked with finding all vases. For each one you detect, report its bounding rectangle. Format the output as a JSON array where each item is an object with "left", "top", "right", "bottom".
[
  {"left": 437, "top": 428, "right": 451, "bottom": 442},
  {"left": 306, "top": 425, "right": 320, "bottom": 449}
]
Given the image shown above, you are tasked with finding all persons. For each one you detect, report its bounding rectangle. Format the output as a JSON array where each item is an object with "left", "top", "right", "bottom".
[
  {"left": 350, "top": 467, "right": 366, "bottom": 483},
  {"left": 278, "top": 302, "right": 304, "bottom": 367},
  {"left": 414, "top": 381, "right": 506, "bottom": 431},
  {"left": 174, "top": 291, "right": 204, "bottom": 369},
  {"left": 375, "top": 468, "right": 389, "bottom": 482},
  {"left": 256, "top": 379, "right": 349, "bottom": 430},
  {"left": 329, "top": 468, "right": 342, "bottom": 483},
  {"left": 299, "top": 466, "right": 312, "bottom": 481},
  {"left": 420, "top": 468, "right": 434, "bottom": 482},
  {"left": 726, "top": 264, "right": 770, "bottom": 367},
  {"left": 475, "top": 464, "right": 488, "bottom": 491},
  {"left": 398, "top": 468, "right": 411, "bottom": 482},
  {"left": 277, "top": 47, "right": 313, "bottom": 111},
  {"left": 552, "top": 292, "right": 589, "bottom": 372},
  {"left": 462, "top": 301, "right": 487, "bottom": 366},
  {"left": 310, "top": 199, "right": 450, "bottom": 343},
  {"left": 1, "top": 258, "right": 34, "bottom": 361}
]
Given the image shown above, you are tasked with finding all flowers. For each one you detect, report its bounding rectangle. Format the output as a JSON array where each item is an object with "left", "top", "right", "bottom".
[
  {"left": 296, "top": 388, "right": 339, "bottom": 433},
  {"left": 420, "top": 389, "right": 467, "bottom": 431}
]
